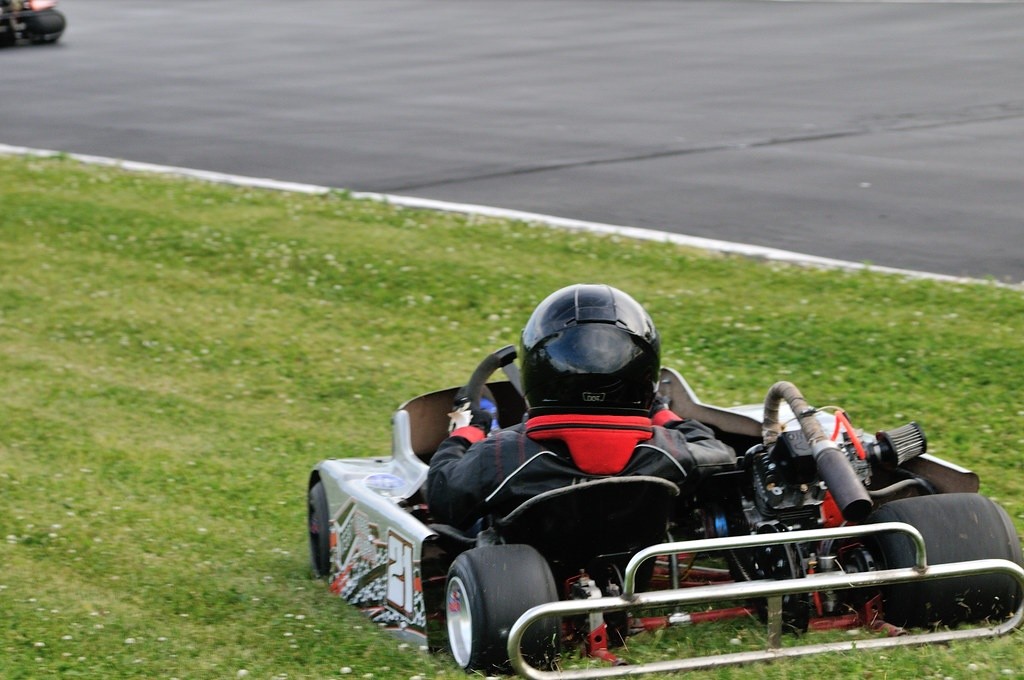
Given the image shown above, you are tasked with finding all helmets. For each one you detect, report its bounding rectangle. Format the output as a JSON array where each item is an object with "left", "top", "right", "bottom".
[{"left": 518, "top": 283, "right": 660, "bottom": 436}]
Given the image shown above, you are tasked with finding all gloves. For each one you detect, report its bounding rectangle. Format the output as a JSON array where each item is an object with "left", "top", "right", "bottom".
[
  {"left": 652, "top": 398, "right": 669, "bottom": 418},
  {"left": 448, "top": 408, "right": 493, "bottom": 444}
]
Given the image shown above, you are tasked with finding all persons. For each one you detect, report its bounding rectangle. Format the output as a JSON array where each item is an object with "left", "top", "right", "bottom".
[{"left": 423, "top": 284, "right": 738, "bottom": 530}]
